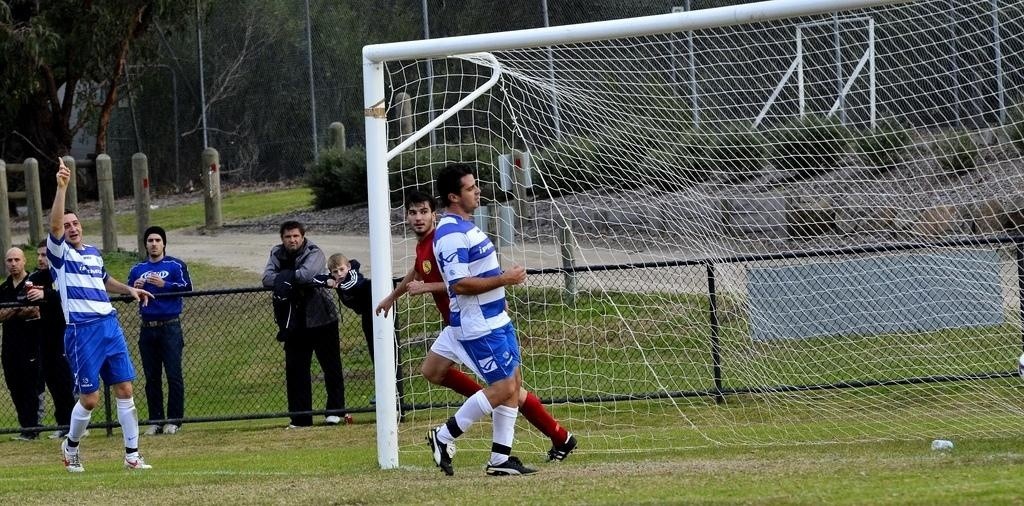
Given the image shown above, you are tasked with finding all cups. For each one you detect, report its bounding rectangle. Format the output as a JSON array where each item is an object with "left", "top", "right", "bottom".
[{"left": 25, "top": 281, "right": 32, "bottom": 294}]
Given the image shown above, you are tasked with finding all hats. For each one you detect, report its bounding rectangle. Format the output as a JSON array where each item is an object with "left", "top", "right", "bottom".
[{"left": 143, "top": 225, "right": 166, "bottom": 246}]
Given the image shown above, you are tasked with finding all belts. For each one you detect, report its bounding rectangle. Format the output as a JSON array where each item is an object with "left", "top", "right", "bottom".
[{"left": 140, "top": 316, "right": 180, "bottom": 329}]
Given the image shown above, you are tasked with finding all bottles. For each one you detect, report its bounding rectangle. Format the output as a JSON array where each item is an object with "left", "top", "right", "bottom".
[{"left": 930, "top": 440, "right": 953, "bottom": 451}]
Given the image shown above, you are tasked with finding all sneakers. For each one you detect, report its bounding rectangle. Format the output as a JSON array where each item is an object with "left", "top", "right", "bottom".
[
  {"left": 123, "top": 455, "right": 153, "bottom": 471},
  {"left": 325, "top": 415, "right": 341, "bottom": 426},
  {"left": 142, "top": 423, "right": 163, "bottom": 437},
  {"left": 161, "top": 423, "right": 180, "bottom": 435},
  {"left": 545, "top": 428, "right": 578, "bottom": 463},
  {"left": 484, "top": 455, "right": 540, "bottom": 478},
  {"left": 10, "top": 429, "right": 39, "bottom": 442},
  {"left": 46, "top": 424, "right": 90, "bottom": 440},
  {"left": 369, "top": 399, "right": 375, "bottom": 405},
  {"left": 423, "top": 425, "right": 457, "bottom": 477},
  {"left": 60, "top": 439, "right": 85, "bottom": 474},
  {"left": 283, "top": 424, "right": 314, "bottom": 431}
]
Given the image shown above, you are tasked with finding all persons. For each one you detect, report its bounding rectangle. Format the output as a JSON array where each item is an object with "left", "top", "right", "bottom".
[
  {"left": 45, "top": 156, "right": 156, "bottom": 472},
  {"left": 312, "top": 252, "right": 405, "bottom": 423},
  {"left": 16, "top": 239, "right": 91, "bottom": 438},
  {"left": 0, "top": 247, "right": 46, "bottom": 441},
  {"left": 121, "top": 225, "right": 194, "bottom": 434},
  {"left": 423, "top": 161, "right": 538, "bottom": 476},
  {"left": 375, "top": 192, "right": 577, "bottom": 464},
  {"left": 260, "top": 220, "right": 345, "bottom": 428}
]
